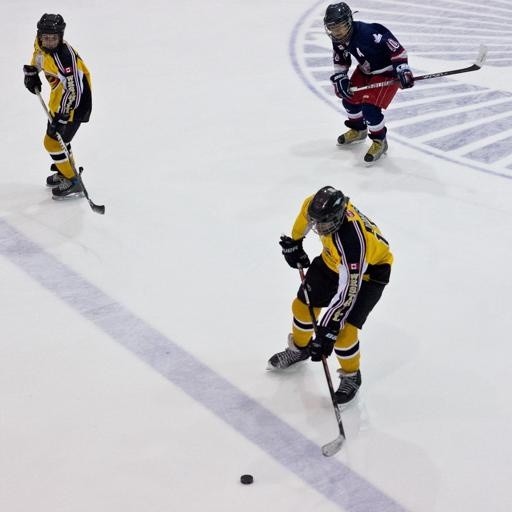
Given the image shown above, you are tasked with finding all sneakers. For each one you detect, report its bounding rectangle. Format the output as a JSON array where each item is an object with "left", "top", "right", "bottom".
[
  {"left": 334, "top": 368, "right": 362, "bottom": 406},
  {"left": 364, "top": 126, "right": 389, "bottom": 162},
  {"left": 337, "top": 120, "right": 368, "bottom": 144},
  {"left": 267, "top": 333, "right": 313, "bottom": 370},
  {"left": 46, "top": 167, "right": 84, "bottom": 195}
]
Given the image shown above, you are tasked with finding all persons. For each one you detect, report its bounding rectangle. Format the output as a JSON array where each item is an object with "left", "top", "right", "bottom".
[
  {"left": 269, "top": 186, "right": 392, "bottom": 404},
  {"left": 324, "top": 3, "right": 415, "bottom": 162},
  {"left": 22, "top": 13, "right": 96, "bottom": 197}
]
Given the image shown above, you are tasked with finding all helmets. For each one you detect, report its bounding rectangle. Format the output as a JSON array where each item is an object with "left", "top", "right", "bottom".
[
  {"left": 36, "top": 12, "right": 66, "bottom": 52},
  {"left": 323, "top": 1, "right": 355, "bottom": 44},
  {"left": 307, "top": 185, "right": 348, "bottom": 236}
]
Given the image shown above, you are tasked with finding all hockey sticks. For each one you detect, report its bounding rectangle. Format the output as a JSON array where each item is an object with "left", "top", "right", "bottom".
[
  {"left": 349, "top": 43, "right": 488, "bottom": 93},
  {"left": 34, "top": 87, "right": 105, "bottom": 215},
  {"left": 297, "top": 262, "right": 347, "bottom": 456}
]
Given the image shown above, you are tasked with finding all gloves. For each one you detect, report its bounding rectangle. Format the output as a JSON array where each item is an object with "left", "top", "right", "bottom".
[
  {"left": 22, "top": 64, "right": 42, "bottom": 94},
  {"left": 279, "top": 235, "right": 311, "bottom": 268},
  {"left": 392, "top": 61, "right": 414, "bottom": 90},
  {"left": 308, "top": 325, "right": 339, "bottom": 362},
  {"left": 329, "top": 69, "right": 354, "bottom": 101},
  {"left": 46, "top": 111, "right": 71, "bottom": 140}
]
